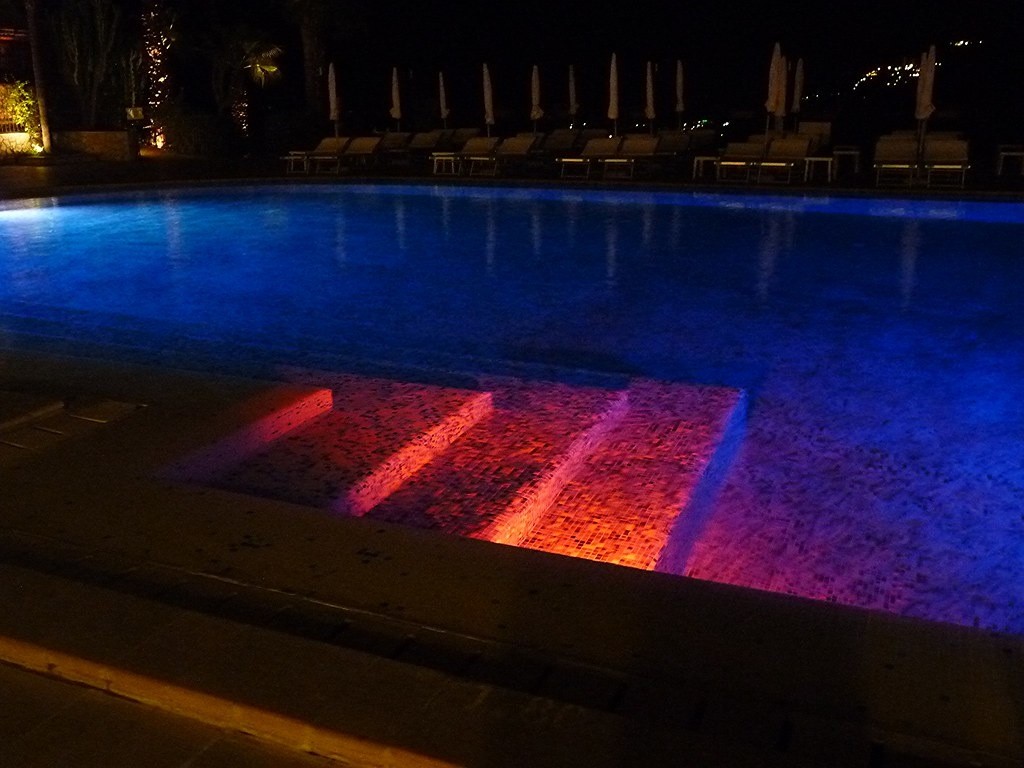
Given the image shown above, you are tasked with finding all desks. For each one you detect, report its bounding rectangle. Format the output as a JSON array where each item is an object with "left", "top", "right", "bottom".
[
  {"left": 833, "top": 145, "right": 861, "bottom": 173},
  {"left": 692, "top": 154, "right": 721, "bottom": 180},
  {"left": 996, "top": 143, "right": 1024, "bottom": 176},
  {"left": 804, "top": 156, "right": 835, "bottom": 183}
]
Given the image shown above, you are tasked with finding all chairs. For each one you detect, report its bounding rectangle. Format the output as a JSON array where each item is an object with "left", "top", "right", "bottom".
[{"left": 279, "top": 128, "right": 714, "bottom": 180}]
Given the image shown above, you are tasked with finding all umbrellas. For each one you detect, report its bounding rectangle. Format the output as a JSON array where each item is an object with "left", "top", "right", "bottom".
[
  {"left": 608, "top": 52, "right": 620, "bottom": 135},
  {"left": 764, "top": 44, "right": 787, "bottom": 141},
  {"left": 390, "top": 67, "right": 401, "bottom": 135},
  {"left": 530, "top": 64, "right": 545, "bottom": 128},
  {"left": 913, "top": 45, "right": 936, "bottom": 164},
  {"left": 325, "top": 63, "right": 339, "bottom": 138},
  {"left": 646, "top": 62, "right": 656, "bottom": 134},
  {"left": 437, "top": 71, "right": 449, "bottom": 127},
  {"left": 566, "top": 63, "right": 580, "bottom": 125},
  {"left": 792, "top": 60, "right": 804, "bottom": 128},
  {"left": 482, "top": 60, "right": 495, "bottom": 135},
  {"left": 676, "top": 59, "right": 685, "bottom": 129}
]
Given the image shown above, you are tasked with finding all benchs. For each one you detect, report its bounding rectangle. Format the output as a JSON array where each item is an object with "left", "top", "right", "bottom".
[
  {"left": 872, "top": 128, "right": 973, "bottom": 190},
  {"left": 714, "top": 120, "right": 832, "bottom": 185}
]
